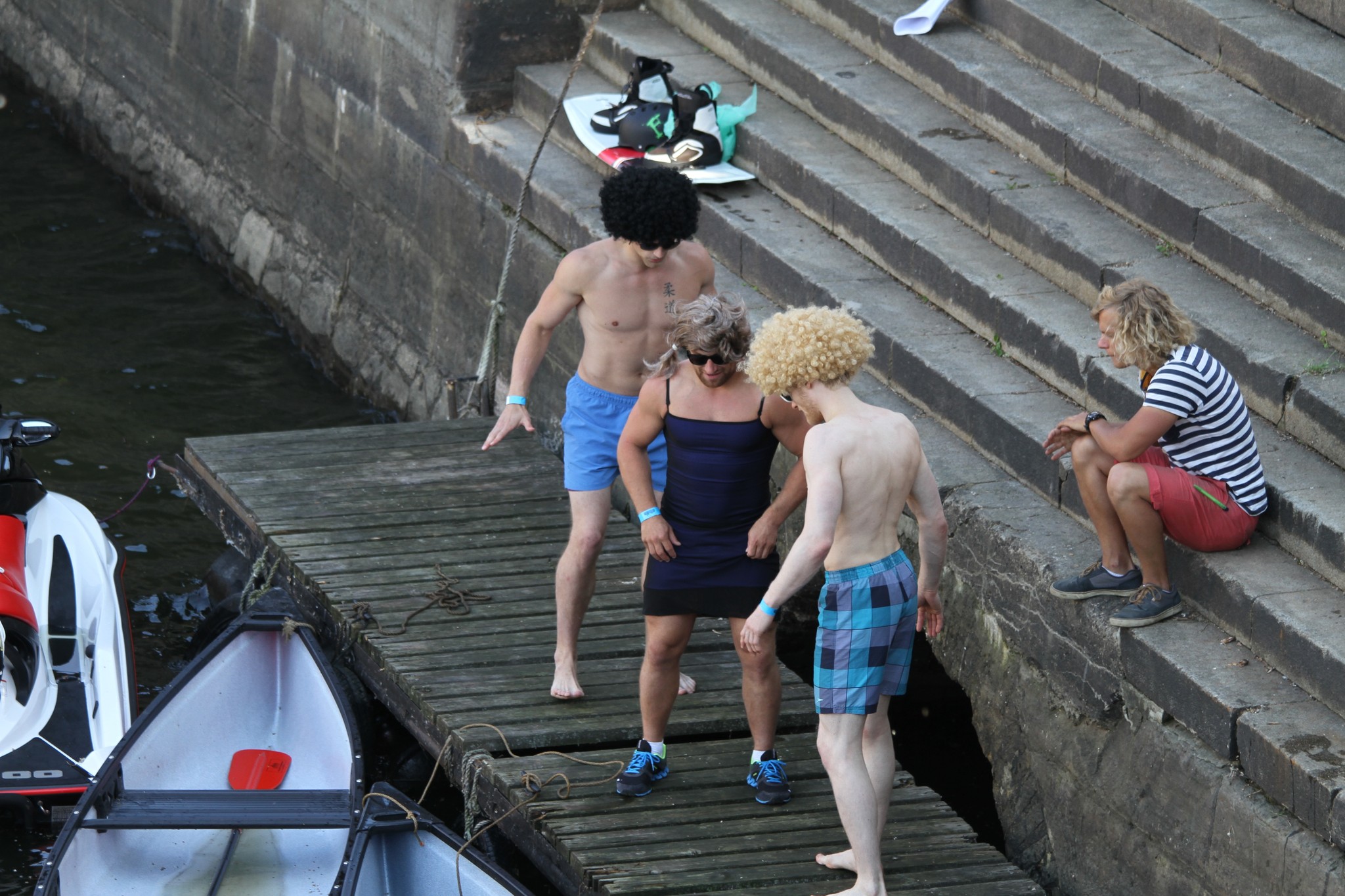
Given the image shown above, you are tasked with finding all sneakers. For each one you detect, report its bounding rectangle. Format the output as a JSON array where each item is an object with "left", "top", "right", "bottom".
[
  {"left": 746, "top": 747, "right": 792, "bottom": 806},
  {"left": 615, "top": 739, "right": 671, "bottom": 798},
  {"left": 1110, "top": 580, "right": 1186, "bottom": 629},
  {"left": 1049, "top": 557, "right": 1144, "bottom": 601}
]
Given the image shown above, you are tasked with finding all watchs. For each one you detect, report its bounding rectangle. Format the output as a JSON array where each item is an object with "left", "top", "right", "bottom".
[{"left": 1083, "top": 411, "right": 1108, "bottom": 433}]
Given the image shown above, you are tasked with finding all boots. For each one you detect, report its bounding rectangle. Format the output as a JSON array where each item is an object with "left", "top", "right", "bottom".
[
  {"left": 645, "top": 83, "right": 724, "bottom": 169},
  {"left": 589, "top": 53, "right": 677, "bottom": 134}
]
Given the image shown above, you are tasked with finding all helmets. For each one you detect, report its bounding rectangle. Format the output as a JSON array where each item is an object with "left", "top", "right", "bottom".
[{"left": 618, "top": 101, "right": 678, "bottom": 153}]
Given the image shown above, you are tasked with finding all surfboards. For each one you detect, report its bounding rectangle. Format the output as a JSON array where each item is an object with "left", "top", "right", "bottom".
[{"left": 562, "top": 92, "right": 755, "bottom": 185}]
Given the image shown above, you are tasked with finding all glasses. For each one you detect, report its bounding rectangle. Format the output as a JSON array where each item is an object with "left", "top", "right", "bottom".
[
  {"left": 638, "top": 238, "right": 681, "bottom": 251},
  {"left": 685, "top": 347, "right": 730, "bottom": 366},
  {"left": 779, "top": 393, "right": 793, "bottom": 403}
]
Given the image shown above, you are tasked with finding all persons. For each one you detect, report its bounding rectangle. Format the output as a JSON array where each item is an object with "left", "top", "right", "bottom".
[
  {"left": 482, "top": 165, "right": 697, "bottom": 701},
  {"left": 740, "top": 302, "right": 950, "bottom": 896},
  {"left": 615, "top": 294, "right": 813, "bottom": 804},
  {"left": 1042, "top": 278, "right": 1269, "bottom": 628}
]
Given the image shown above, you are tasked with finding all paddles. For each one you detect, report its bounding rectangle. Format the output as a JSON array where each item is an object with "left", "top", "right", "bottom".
[{"left": 206, "top": 749, "right": 291, "bottom": 896}]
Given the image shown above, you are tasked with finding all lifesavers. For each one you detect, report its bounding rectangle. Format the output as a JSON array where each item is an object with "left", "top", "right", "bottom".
[
  {"left": 206, "top": 550, "right": 253, "bottom": 621},
  {"left": 335, "top": 660, "right": 374, "bottom": 728}
]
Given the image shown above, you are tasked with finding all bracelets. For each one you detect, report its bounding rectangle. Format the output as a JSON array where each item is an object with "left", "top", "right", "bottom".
[
  {"left": 506, "top": 396, "right": 527, "bottom": 406},
  {"left": 638, "top": 506, "right": 661, "bottom": 523},
  {"left": 760, "top": 598, "right": 779, "bottom": 616}
]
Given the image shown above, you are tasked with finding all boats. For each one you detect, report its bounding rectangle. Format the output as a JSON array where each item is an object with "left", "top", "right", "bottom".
[
  {"left": 32, "top": 584, "right": 368, "bottom": 896},
  {"left": 339, "top": 778, "right": 533, "bottom": 896}
]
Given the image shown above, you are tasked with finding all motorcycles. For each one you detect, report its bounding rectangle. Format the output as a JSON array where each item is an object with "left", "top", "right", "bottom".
[{"left": 0, "top": 408, "right": 132, "bottom": 828}]
